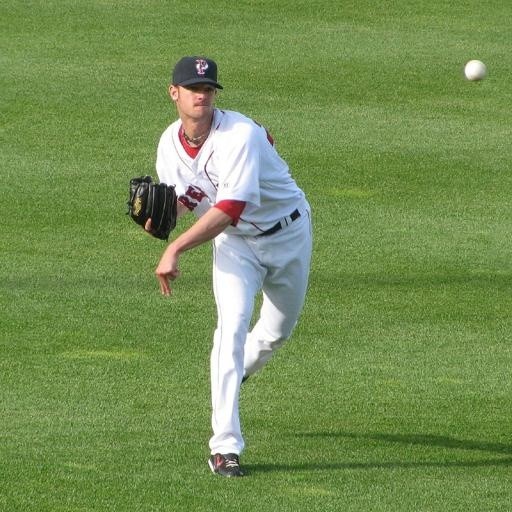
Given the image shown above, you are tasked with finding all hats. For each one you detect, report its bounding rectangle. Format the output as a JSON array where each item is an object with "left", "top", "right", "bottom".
[{"left": 173, "top": 57, "right": 223, "bottom": 90}]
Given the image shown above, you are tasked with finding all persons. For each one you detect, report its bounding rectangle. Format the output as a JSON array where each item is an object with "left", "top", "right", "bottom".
[{"left": 126, "top": 53, "right": 312, "bottom": 481}]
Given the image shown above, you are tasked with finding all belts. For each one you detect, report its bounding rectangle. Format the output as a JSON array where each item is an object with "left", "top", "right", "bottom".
[{"left": 259, "top": 209, "right": 297, "bottom": 239}]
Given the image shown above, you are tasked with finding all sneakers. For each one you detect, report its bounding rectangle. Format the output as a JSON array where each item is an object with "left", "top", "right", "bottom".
[{"left": 209, "top": 452, "right": 241, "bottom": 479}]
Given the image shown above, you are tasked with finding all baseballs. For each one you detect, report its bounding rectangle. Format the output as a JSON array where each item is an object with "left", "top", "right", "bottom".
[{"left": 465, "top": 60, "right": 487, "bottom": 81}]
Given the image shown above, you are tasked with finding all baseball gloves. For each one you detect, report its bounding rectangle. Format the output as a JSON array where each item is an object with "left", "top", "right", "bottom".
[{"left": 126, "top": 176, "right": 177, "bottom": 241}]
